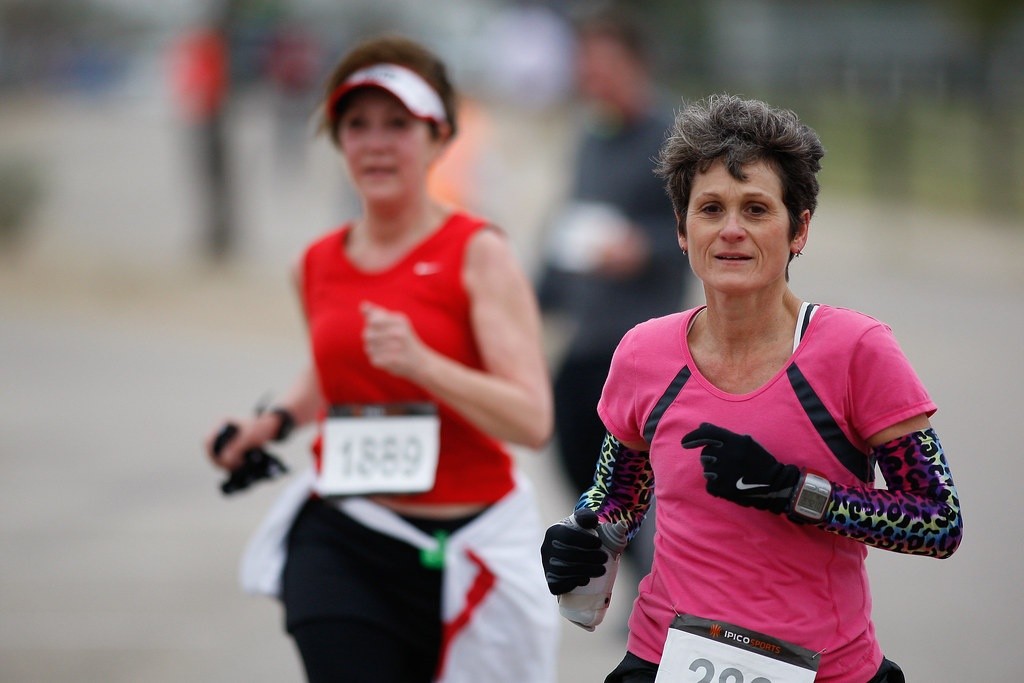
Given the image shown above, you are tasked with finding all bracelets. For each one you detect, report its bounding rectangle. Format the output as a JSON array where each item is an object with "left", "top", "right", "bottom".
[{"left": 261, "top": 405, "right": 294, "bottom": 441}]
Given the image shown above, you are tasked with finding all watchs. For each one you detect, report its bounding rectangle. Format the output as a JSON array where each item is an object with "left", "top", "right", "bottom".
[{"left": 784, "top": 465, "right": 833, "bottom": 527}]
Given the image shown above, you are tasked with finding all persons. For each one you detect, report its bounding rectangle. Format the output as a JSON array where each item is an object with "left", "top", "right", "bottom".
[
  {"left": 541, "top": 98, "right": 962, "bottom": 683},
  {"left": 174, "top": 12, "right": 233, "bottom": 259},
  {"left": 205, "top": 40, "right": 560, "bottom": 683},
  {"left": 530, "top": 31, "right": 698, "bottom": 587}
]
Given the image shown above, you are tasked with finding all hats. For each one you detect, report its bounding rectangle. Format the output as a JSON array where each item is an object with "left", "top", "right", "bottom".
[{"left": 309, "top": 60, "right": 447, "bottom": 135}]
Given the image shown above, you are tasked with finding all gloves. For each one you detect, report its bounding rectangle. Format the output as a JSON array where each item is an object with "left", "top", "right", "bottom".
[
  {"left": 681, "top": 422, "right": 800, "bottom": 516},
  {"left": 541, "top": 508, "right": 608, "bottom": 595}
]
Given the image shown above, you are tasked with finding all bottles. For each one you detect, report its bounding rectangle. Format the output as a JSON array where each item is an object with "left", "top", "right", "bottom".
[{"left": 558, "top": 513, "right": 628, "bottom": 633}]
